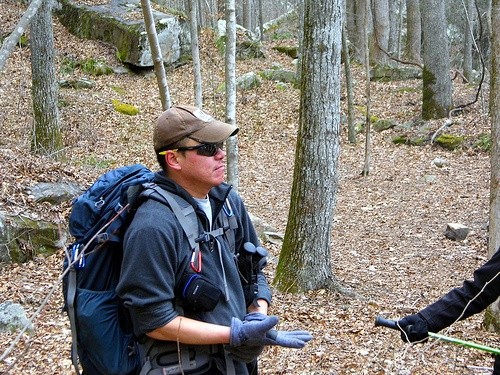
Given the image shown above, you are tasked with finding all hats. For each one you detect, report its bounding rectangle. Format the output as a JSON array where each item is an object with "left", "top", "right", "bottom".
[{"left": 154, "top": 105, "right": 238, "bottom": 149}]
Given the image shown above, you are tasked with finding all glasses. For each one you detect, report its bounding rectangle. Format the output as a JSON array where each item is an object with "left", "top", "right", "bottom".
[{"left": 159, "top": 141, "right": 224, "bottom": 157}]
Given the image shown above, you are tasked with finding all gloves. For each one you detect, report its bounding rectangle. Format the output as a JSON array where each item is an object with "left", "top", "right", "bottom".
[
  {"left": 229, "top": 317, "right": 312, "bottom": 348},
  {"left": 224, "top": 312, "right": 268, "bottom": 364},
  {"left": 397, "top": 315, "right": 429, "bottom": 344}
]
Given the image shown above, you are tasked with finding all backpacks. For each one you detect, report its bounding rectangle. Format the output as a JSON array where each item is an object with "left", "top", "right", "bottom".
[{"left": 58, "top": 163, "right": 259, "bottom": 375}]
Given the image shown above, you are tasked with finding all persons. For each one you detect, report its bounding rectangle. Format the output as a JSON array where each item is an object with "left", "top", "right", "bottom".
[
  {"left": 396, "top": 246, "right": 500, "bottom": 375},
  {"left": 116, "top": 105, "right": 312, "bottom": 375}
]
